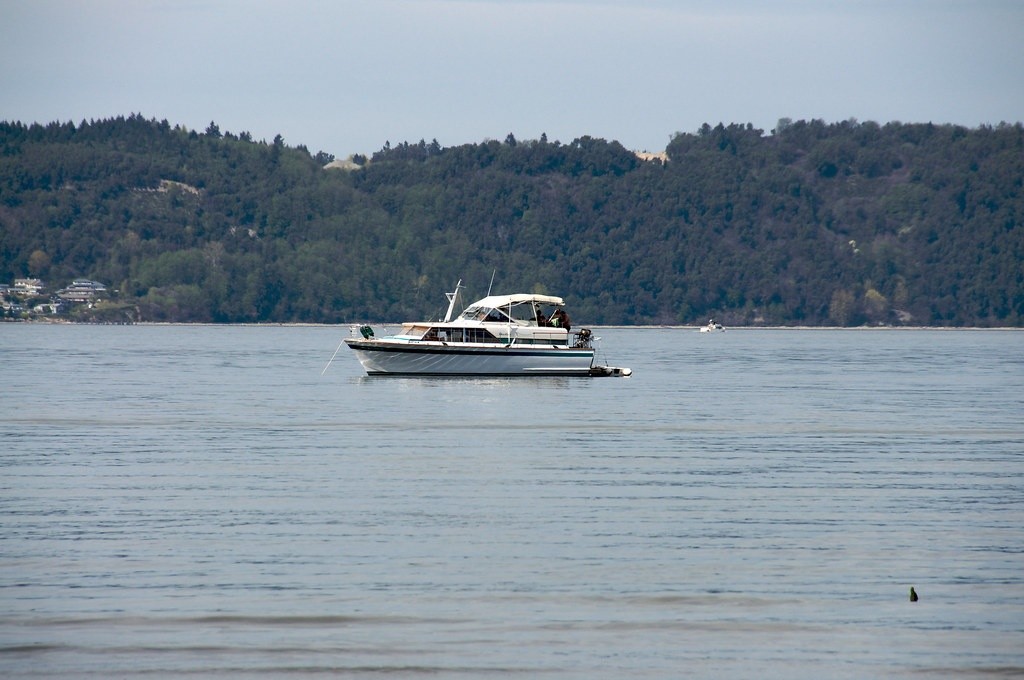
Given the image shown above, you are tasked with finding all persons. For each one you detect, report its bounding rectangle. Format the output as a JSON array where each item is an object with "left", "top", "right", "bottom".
[
  {"left": 485, "top": 308, "right": 490, "bottom": 321},
  {"left": 553, "top": 309, "right": 571, "bottom": 333},
  {"left": 536, "top": 310, "right": 545, "bottom": 326},
  {"left": 500, "top": 313, "right": 509, "bottom": 322}
]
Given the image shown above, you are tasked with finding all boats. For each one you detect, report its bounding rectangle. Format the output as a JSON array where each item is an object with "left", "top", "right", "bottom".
[
  {"left": 699, "top": 323, "right": 726, "bottom": 332},
  {"left": 342, "top": 265, "right": 633, "bottom": 378}
]
